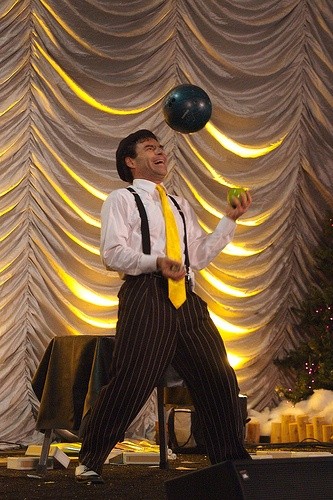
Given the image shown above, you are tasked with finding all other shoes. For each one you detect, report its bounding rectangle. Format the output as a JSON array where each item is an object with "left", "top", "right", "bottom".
[{"left": 75, "top": 465, "right": 104, "bottom": 484}]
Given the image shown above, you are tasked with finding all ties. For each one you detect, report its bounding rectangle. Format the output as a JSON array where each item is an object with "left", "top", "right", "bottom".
[{"left": 156, "top": 183, "right": 187, "bottom": 310}]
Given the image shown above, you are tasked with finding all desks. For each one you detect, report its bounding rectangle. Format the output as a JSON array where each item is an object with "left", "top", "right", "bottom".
[{"left": 31, "top": 335, "right": 168, "bottom": 474}]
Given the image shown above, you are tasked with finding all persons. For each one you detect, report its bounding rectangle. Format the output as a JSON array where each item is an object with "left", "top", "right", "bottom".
[{"left": 75, "top": 128, "right": 251, "bottom": 484}]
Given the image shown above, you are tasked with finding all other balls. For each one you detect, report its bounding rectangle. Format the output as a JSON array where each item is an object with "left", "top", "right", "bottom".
[
  {"left": 172, "top": 263, "right": 181, "bottom": 272},
  {"left": 162, "top": 84, "right": 212, "bottom": 134},
  {"left": 227, "top": 188, "right": 248, "bottom": 209}
]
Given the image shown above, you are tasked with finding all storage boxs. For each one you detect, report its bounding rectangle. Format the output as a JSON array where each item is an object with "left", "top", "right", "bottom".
[{"left": 8, "top": 445, "right": 160, "bottom": 470}]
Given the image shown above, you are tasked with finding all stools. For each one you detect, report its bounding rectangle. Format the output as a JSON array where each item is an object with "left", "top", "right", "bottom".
[{"left": 164, "top": 393, "right": 248, "bottom": 454}]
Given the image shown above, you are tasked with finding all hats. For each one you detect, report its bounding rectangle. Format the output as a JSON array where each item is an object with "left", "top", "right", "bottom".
[{"left": 115, "top": 128, "right": 157, "bottom": 180}]
